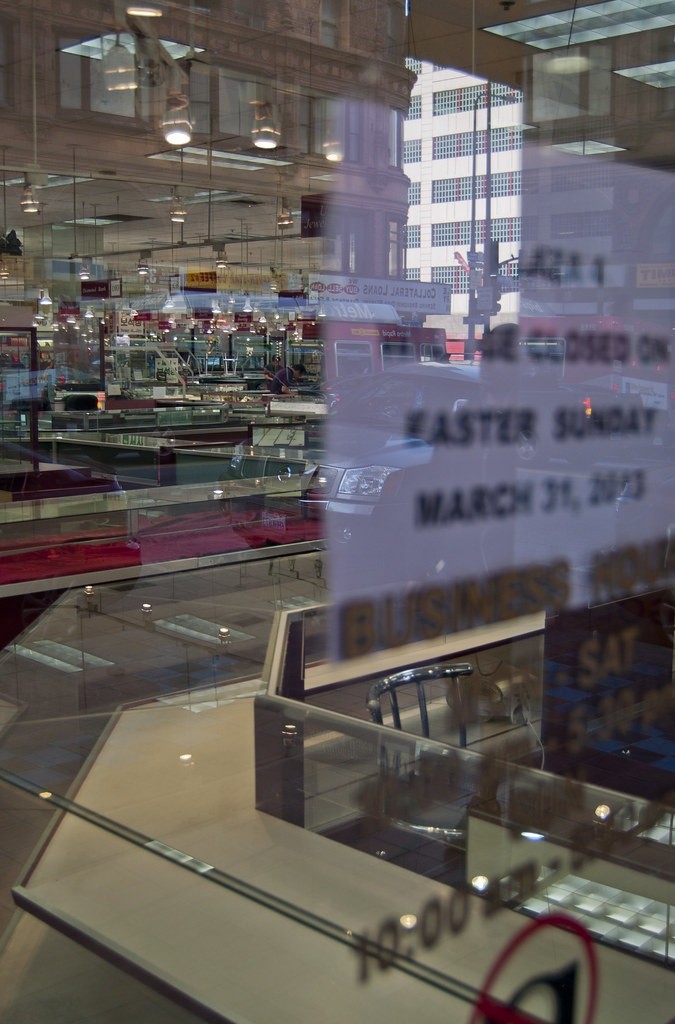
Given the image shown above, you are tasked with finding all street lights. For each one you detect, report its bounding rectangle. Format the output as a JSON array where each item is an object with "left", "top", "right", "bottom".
[{"left": 467, "top": 94, "right": 519, "bottom": 360}]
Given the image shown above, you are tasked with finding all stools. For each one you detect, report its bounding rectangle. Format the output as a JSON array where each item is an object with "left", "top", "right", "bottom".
[{"left": 367, "top": 662, "right": 489, "bottom": 841}]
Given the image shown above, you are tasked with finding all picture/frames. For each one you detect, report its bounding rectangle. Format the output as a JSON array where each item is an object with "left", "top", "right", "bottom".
[
  {"left": 169, "top": 276, "right": 182, "bottom": 295},
  {"left": 110, "top": 278, "right": 122, "bottom": 297}
]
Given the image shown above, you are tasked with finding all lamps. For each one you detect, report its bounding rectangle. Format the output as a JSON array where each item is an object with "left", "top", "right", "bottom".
[
  {"left": 79, "top": 264, "right": 89, "bottom": 281},
  {"left": 215, "top": 250, "right": 229, "bottom": 268},
  {"left": 137, "top": 257, "right": 150, "bottom": 275},
  {"left": 104, "top": 12, "right": 196, "bottom": 144},
  {"left": 32, "top": 289, "right": 326, "bottom": 343},
  {"left": 248, "top": 94, "right": 283, "bottom": 148},
  {"left": 169, "top": 194, "right": 187, "bottom": 223},
  {"left": 21, "top": 185, "right": 40, "bottom": 214},
  {"left": 277, "top": 198, "right": 293, "bottom": 226}
]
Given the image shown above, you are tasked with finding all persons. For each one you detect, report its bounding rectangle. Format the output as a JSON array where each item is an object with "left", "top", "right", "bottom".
[
  {"left": 264, "top": 355, "right": 282, "bottom": 391},
  {"left": 271, "top": 364, "right": 306, "bottom": 394}
]
